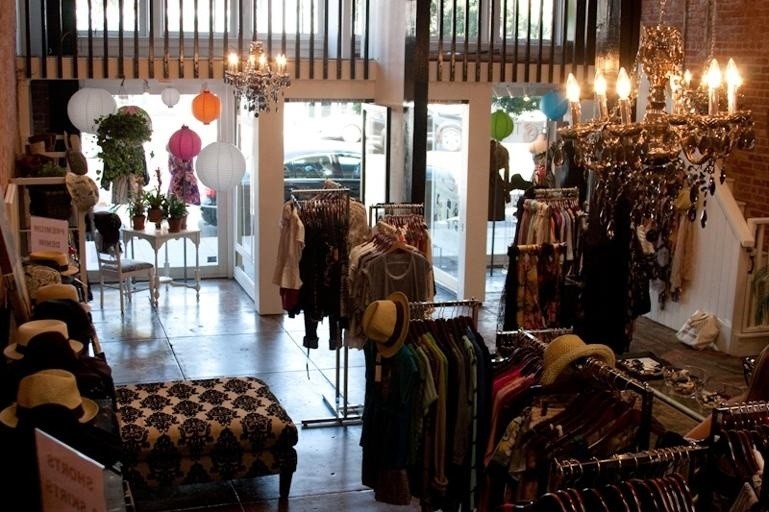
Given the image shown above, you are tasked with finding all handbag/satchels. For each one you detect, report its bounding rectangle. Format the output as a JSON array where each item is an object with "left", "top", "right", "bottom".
[{"left": 675, "top": 310, "right": 719, "bottom": 351}]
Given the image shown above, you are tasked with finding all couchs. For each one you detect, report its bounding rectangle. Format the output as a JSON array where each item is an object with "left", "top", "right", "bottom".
[{"left": 115, "top": 377, "right": 298, "bottom": 501}]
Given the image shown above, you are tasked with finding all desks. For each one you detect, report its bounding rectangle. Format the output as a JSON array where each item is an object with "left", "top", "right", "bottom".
[{"left": 121, "top": 227, "right": 202, "bottom": 308}]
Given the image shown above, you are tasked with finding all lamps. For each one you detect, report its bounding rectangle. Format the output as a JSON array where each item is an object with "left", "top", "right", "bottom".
[
  {"left": 557, "top": 1, "right": 757, "bottom": 180},
  {"left": 223, "top": 0, "right": 291, "bottom": 91}
]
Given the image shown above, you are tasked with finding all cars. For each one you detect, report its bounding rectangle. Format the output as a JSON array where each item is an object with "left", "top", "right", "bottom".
[
  {"left": 316, "top": 109, "right": 362, "bottom": 144},
  {"left": 198, "top": 150, "right": 459, "bottom": 235},
  {"left": 364, "top": 107, "right": 464, "bottom": 153}
]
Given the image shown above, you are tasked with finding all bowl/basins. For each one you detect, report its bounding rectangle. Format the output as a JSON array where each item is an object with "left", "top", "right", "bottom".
[
  {"left": 697, "top": 382, "right": 744, "bottom": 413},
  {"left": 661, "top": 366, "right": 707, "bottom": 396}
]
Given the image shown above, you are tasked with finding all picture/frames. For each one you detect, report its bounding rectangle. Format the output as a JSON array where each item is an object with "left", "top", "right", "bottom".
[{"left": 615, "top": 351, "right": 672, "bottom": 380}]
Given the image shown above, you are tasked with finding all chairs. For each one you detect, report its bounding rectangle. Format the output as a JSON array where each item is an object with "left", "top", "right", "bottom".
[{"left": 89, "top": 212, "right": 155, "bottom": 314}]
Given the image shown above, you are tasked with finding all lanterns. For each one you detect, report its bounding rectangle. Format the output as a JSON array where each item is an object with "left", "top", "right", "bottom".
[
  {"left": 192, "top": 90, "right": 220, "bottom": 126},
  {"left": 539, "top": 91, "right": 568, "bottom": 123},
  {"left": 196, "top": 141, "right": 246, "bottom": 193},
  {"left": 161, "top": 88, "right": 180, "bottom": 109},
  {"left": 67, "top": 87, "right": 116, "bottom": 134},
  {"left": 490, "top": 108, "right": 514, "bottom": 143},
  {"left": 169, "top": 125, "right": 201, "bottom": 158}
]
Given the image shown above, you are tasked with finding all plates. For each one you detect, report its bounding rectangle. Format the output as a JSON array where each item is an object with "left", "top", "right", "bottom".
[{"left": 615, "top": 351, "right": 682, "bottom": 380}]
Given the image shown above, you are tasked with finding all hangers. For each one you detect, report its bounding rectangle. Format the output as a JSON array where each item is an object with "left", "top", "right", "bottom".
[
  {"left": 496, "top": 326, "right": 706, "bottom": 424},
  {"left": 409, "top": 297, "right": 482, "bottom": 332},
  {"left": 508, "top": 242, "right": 568, "bottom": 329},
  {"left": 369, "top": 203, "right": 425, "bottom": 244},
  {"left": 289, "top": 179, "right": 365, "bottom": 428},
  {"left": 536, "top": 400, "right": 768, "bottom": 512},
  {"left": 375, "top": 220, "right": 402, "bottom": 238},
  {"left": 534, "top": 187, "right": 579, "bottom": 206}
]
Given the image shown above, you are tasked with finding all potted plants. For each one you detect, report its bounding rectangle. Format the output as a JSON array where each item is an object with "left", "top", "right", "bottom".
[
  {"left": 181, "top": 206, "right": 188, "bottom": 229},
  {"left": 159, "top": 194, "right": 191, "bottom": 232},
  {"left": 125, "top": 189, "right": 146, "bottom": 231},
  {"left": 92, "top": 110, "right": 154, "bottom": 204},
  {"left": 140, "top": 187, "right": 170, "bottom": 222}
]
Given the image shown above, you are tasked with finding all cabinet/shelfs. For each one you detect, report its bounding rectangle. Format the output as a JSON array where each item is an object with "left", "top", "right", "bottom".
[{"left": 9, "top": 150, "right": 89, "bottom": 304}]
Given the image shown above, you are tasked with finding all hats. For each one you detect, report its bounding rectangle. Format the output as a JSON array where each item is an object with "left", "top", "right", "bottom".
[
  {"left": 65, "top": 171, "right": 99, "bottom": 211},
  {"left": 361, "top": 291, "right": 410, "bottom": 359},
  {"left": 675, "top": 187, "right": 704, "bottom": 215},
  {"left": 7, "top": 331, "right": 98, "bottom": 380},
  {"left": 751, "top": 264, "right": 768, "bottom": 323},
  {"left": 4, "top": 320, "right": 83, "bottom": 359},
  {"left": 21, "top": 249, "right": 80, "bottom": 275},
  {"left": 94, "top": 211, "right": 121, "bottom": 242},
  {"left": 66, "top": 147, "right": 87, "bottom": 175},
  {"left": 530, "top": 140, "right": 553, "bottom": 155},
  {"left": 31, "top": 297, "right": 89, "bottom": 339},
  {"left": 16, "top": 264, "right": 63, "bottom": 299},
  {"left": 33, "top": 283, "right": 91, "bottom": 314},
  {"left": 4, "top": 403, "right": 122, "bottom": 480},
  {"left": 541, "top": 334, "right": 616, "bottom": 386},
  {"left": 1, "top": 369, "right": 100, "bottom": 427}
]
